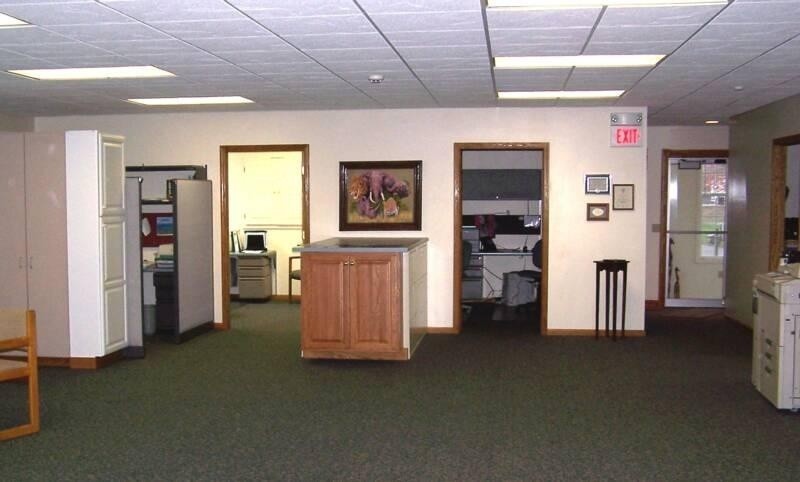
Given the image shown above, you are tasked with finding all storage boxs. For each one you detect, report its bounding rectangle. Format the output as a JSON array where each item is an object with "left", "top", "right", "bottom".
[{"left": 459, "top": 225, "right": 484, "bottom": 301}]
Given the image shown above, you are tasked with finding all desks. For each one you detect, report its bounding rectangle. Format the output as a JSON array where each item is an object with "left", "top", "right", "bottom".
[
  {"left": 593, "top": 258, "right": 630, "bottom": 341},
  {"left": 228, "top": 249, "right": 276, "bottom": 301}
]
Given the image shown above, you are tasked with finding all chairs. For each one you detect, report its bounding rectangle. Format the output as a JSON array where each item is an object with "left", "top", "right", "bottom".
[
  {"left": 289, "top": 256, "right": 301, "bottom": 304},
  {"left": 0, "top": 307, "right": 41, "bottom": 442}
]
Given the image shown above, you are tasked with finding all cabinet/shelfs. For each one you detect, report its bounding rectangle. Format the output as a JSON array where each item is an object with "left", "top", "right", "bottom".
[
  {"left": 140, "top": 195, "right": 179, "bottom": 274},
  {"left": 297, "top": 251, "right": 411, "bottom": 363},
  {"left": 0, "top": 131, "right": 71, "bottom": 360}
]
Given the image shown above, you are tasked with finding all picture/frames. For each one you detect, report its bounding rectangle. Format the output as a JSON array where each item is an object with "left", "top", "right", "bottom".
[
  {"left": 612, "top": 183, "right": 635, "bottom": 210},
  {"left": 584, "top": 173, "right": 610, "bottom": 195},
  {"left": 586, "top": 203, "right": 610, "bottom": 222},
  {"left": 338, "top": 159, "right": 423, "bottom": 232}
]
